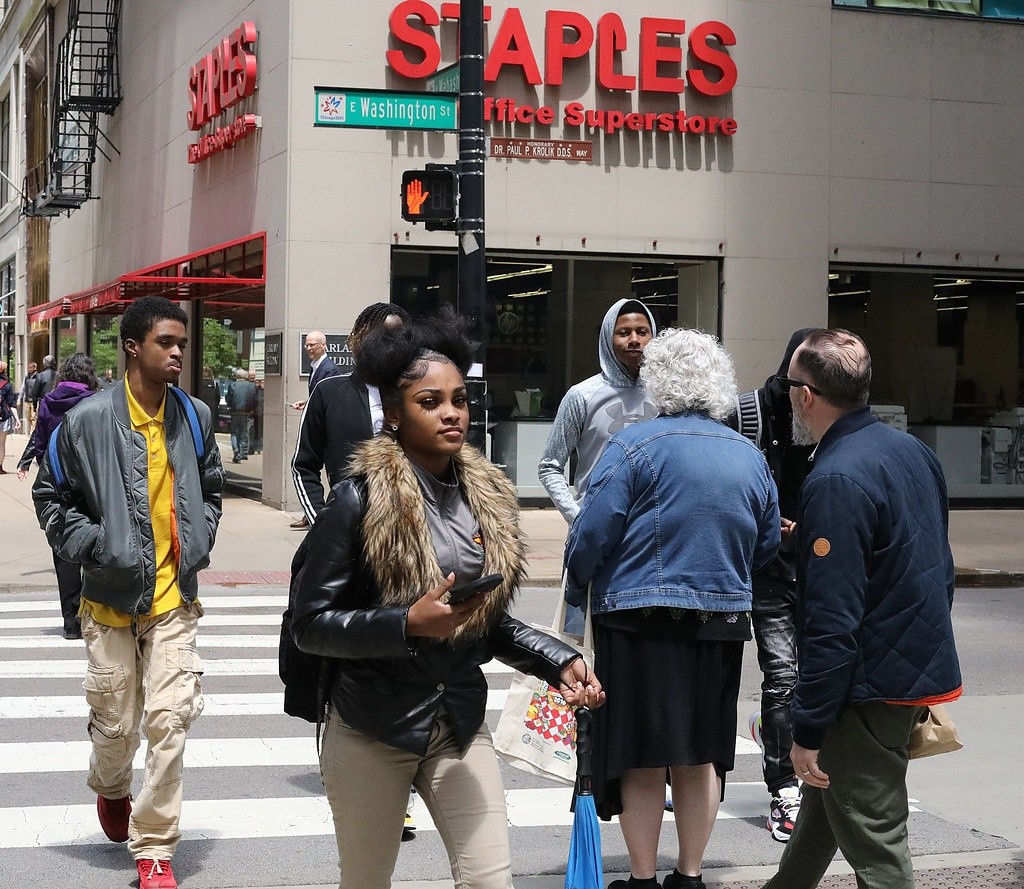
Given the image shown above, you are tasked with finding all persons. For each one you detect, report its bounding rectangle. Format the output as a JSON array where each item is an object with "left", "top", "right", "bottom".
[
  {"left": 17, "top": 352, "right": 101, "bottom": 640},
  {"left": 31, "top": 355, "right": 58, "bottom": 465},
  {"left": 17, "top": 361, "right": 39, "bottom": 435},
  {"left": 201, "top": 366, "right": 220, "bottom": 431},
  {"left": 31, "top": 296, "right": 226, "bottom": 887},
  {"left": 288, "top": 318, "right": 608, "bottom": 889},
  {"left": 217, "top": 420, "right": 229, "bottom": 433},
  {"left": 101, "top": 369, "right": 118, "bottom": 388},
  {"left": 225, "top": 369, "right": 264, "bottom": 463},
  {"left": 539, "top": 298, "right": 676, "bottom": 813},
  {"left": 0, "top": 361, "right": 21, "bottom": 474},
  {"left": 763, "top": 328, "right": 963, "bottom": 889},
  {"left": 725, "top": 329, "right": 825, "bottom": 844},
  {"left": 561, "top": 327, "right": 783, "bottom": 889},
  {"left": 291, "top": 301, "right": 428, "bottom": 833}
]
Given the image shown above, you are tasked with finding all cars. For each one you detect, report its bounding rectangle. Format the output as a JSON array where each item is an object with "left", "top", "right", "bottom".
[{"left": 216, "top": 377, "right": 235, "bottom": 433}]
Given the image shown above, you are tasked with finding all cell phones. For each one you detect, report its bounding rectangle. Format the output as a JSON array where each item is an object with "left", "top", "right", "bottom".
[{"left": 447, "top": 573, "right": 504, "bottom": 606}]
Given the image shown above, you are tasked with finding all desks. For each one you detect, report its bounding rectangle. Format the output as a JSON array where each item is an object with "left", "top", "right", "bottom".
[
  {"left": 908, "top": 422, "right": 1023, "bottom": 486},
  {"left": 492, "top": 417, "right": 570, "bottom": 487}
]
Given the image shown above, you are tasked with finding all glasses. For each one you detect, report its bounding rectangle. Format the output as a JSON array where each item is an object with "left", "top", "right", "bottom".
[
  {"left": 303, "top": 342, "right": 321, "bottom": 348},
  {"left": 776, "top": 374, "right": 822, "bottom": 396}
]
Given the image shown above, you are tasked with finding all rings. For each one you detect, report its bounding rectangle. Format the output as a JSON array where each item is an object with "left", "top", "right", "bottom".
[{"left": 802, "top": 770, "right": 810, "bottom": 776}]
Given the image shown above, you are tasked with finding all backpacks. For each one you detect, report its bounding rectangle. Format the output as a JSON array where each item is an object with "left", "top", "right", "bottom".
[
  {"left": 0, "top": 379, "right": 11, "bottom": 418},
  {"left": 278, "top": 476, "right": 370, "bottom": 724}
]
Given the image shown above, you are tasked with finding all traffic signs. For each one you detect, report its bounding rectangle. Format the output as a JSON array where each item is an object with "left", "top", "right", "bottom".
[{"left": 313, "top": 86, "right": 459, "bottom": 132}]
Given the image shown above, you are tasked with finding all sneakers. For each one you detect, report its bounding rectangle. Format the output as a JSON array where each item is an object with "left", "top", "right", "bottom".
[
  {"left": 767, "top": 785, "right": 804, "bottom": 840},
  {"left": 98, "top": 792, "right": 133, "bottom": 842},
  {"left": 136, "top": 858, "right": 178, "bottom": 889},
  {"left": 748, "top": 710, "right": 768, "bottom": 774}
]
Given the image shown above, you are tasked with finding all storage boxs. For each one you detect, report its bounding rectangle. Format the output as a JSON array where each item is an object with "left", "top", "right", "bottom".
[{"left": 989, "top": 407, "right": 1024, "bottom": 424}]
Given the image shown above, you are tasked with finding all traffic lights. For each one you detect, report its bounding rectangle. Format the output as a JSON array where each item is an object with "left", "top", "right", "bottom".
[{"left": 399, "top": 170, "right": 458, "bottom": 225}]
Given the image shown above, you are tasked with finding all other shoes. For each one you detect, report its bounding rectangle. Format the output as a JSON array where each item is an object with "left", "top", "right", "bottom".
[
  {"left": 0, "top": 466, "right": 6, "bottom": 473},
  {"left": 290, "top": 514, "right": 311, "bottom": 530},
  {"left": 232, "top": 450, "right": 242, "bottom": 462},
  {"left": 608, "top": 874, "right": 707, "bottom": 889},
  {"left": 63, "top": 620, "right": 82, "bottom": 638}
]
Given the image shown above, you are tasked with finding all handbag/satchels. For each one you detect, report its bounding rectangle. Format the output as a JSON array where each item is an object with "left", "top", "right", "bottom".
[{"left": 493, "top": 568, "right": 595, "bottom": 786}]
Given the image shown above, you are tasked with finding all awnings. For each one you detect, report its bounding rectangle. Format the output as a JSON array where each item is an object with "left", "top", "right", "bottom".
[{"left": 27, "top": 273, "right": 267, "bottom": 325}]
